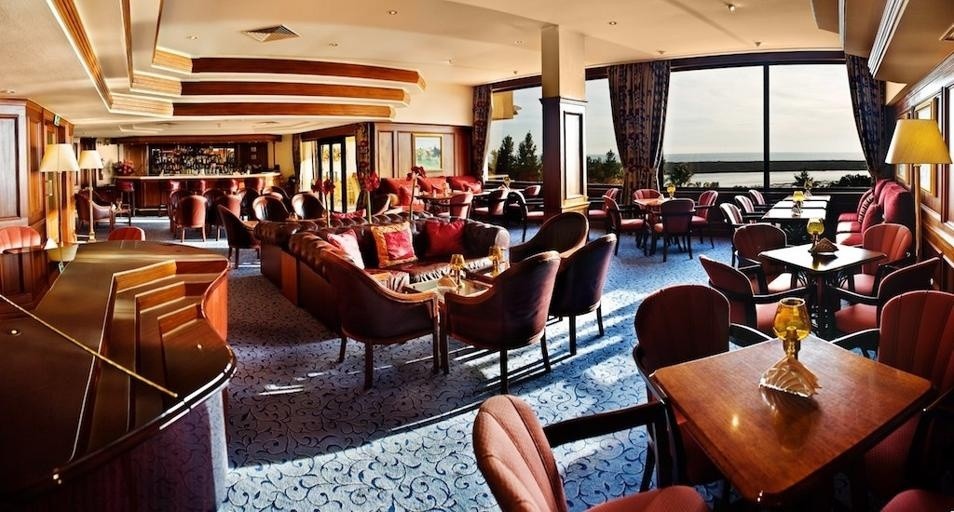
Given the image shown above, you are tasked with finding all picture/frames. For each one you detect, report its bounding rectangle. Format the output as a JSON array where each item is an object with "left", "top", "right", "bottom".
[
  {"left": 913, "top": 96, "right": 939, "bottom": 196},
  {"left": 894, "top": 113, "right": 910, "bottom": 185},
  {"left": 410, "top": 132, "right": 445, "bottom": 172}
]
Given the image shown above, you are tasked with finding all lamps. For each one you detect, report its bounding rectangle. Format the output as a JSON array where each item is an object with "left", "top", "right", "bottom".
[
  {"left": 884, "top": 118, "right": 952, "bottom": 262},
  {"left": 77, "top": 150, "right": 104, "bottom": 242},
  {"left": 40, "top": 142, "right": 80, "bottom": 247}
]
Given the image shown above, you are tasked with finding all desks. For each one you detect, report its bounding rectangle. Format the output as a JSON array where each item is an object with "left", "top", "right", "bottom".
[{"left": 646, "top": 332, "right": 937, "bottom": 511}]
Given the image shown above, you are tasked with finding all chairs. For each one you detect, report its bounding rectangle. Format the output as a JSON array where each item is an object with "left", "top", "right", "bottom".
[
  {"left": 828, "top": 290, "right": 954, "bottom": 511},
  {"left": 881, "top": 471, "right": 954, "bottom": 512},
  {"left": 474, "top": 395, "right": 705, "bottom": 511},
  {"left": 633, "top": 285, "right": 774, "bottom": 492}
]
